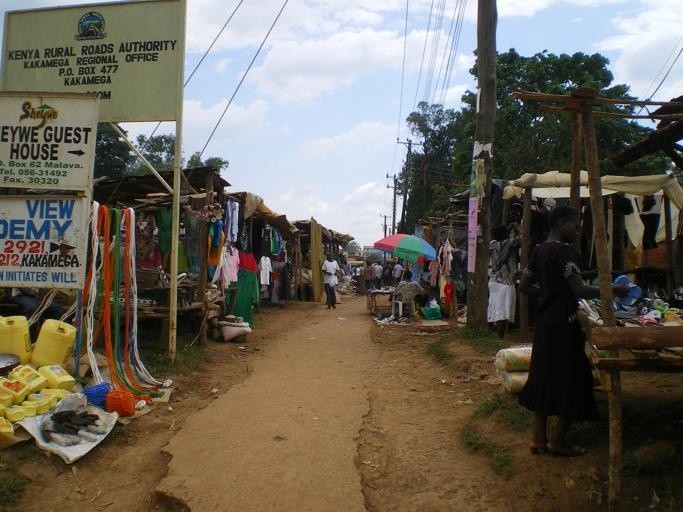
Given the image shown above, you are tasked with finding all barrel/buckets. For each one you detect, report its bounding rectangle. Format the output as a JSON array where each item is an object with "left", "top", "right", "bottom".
[{"left": 0, "top": 314, "right": 77, "bottom": 434}]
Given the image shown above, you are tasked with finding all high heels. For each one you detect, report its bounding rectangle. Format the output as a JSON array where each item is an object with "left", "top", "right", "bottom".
[{"left": 529, "top": 441, "right": 588, "bottom": 457}]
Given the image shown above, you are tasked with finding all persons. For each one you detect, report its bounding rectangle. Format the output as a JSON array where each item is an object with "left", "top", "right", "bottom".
[
  {"left": 320, "top": 253, "right": 339, "bottom": 310},
  {"left": 518, "top": 206, "right": 629, "bottom": 455},
  {"left": 350, "top": 261, "right": 397, "bottom": 290},
  {"left": 394, "top": 270, "right": 425, "bottom": 313},
  {"left": 486, "top": 223, "right": 522, "bottom": 340}
]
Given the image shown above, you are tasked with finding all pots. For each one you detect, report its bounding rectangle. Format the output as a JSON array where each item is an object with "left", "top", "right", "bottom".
[{"left": 0, "top": 353, "right": 20, "bottom": 374}]
